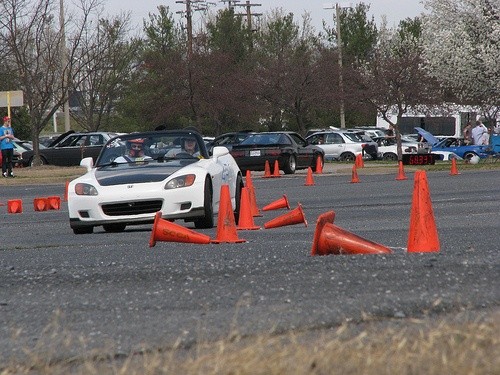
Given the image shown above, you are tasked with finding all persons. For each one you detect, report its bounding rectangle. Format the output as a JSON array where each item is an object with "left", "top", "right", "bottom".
[
  {"left": 463, "top": 121, "right": 491, "bottom": 145},
  {"left": 386, "top": 124, "right": 394, "bottom": 136},
  {"left": 164, "top": 134, "right": 200, "bottom": 158},
  {"left": 113, "top": 139, "right": 152, "bottom": 163},
  {"left": 420, "top": 136, "right": 429, "bottom": 149},
  {"left": 0, "top": 117, "right": 15, "bottom": 178}
]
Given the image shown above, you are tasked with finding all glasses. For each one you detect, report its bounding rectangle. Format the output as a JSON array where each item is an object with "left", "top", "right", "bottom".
[{"left": 133, "top": 145, "right": 141, "bottom": 148}]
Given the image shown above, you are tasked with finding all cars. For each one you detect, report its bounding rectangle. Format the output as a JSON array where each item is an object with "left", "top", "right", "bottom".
[
  {"left": 304, "top": 130, "right": 378, "bottom": 163},
  {"left": 230, "top": 131, "right": 325, "bottom": 175},
  {"left": 0, "top": 126, "right": 500, "bottom": 168}
]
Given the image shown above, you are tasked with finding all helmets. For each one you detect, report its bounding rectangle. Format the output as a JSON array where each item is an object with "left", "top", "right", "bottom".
[
  {"left": 127, "top": 137, "right": 144, "bottom": 157},
  {"left": 180, "top": 133, "right": 198, "bottom": 151}
]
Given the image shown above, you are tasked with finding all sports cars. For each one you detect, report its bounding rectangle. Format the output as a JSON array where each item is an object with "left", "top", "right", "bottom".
[{"left": 67, "top": 128, "right": 245, "bottom": 234}]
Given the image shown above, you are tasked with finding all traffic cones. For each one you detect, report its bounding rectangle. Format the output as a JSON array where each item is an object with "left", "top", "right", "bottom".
[
  {"left": 304, "top": 165, "right": 317, "bottom": 186},
  {"left": 7, "top": 200, "right": 23, "bottom": 213},
  {"left": 354, "top": 154, "right": 364, "bottom": 168},
  {"left": 264, "top": 201, "right": 308, "bottom": 229},
  {"left": 270, "top": 160, "right": 282, "bottom": 177},
  {"left": 47, "top": 196, "right": 61, "bottom": 210},
  {"left": 350, "top": 164, "right": 361, "bottom": 183},
  {"left": 236, "top": 186, "right": 261, "bottom": 230},
  {"left": 209, "top": 184, "right": 247, "bottom": 243},
  {"left": 311, "top": 209, "right": 392, "bottom": 256},
  {"left": 448, "top": 157, "right": 460, "bottom": 175},
  {"left": 314, "top": 156, "right": 323, "bottom": 174},
  {"left": 394, "top": 159, "right": 408, "bottom": 180},
  {"left": 261, "top": 193, "right": 291, "bottom": 211},
  {"left": 245, "top": 169, "right": 262, "bottom": 217},
  {"left": 33, "top": 197, "right": 48, "bottom": 211},
  {"left": 261, "top": 160, "right": 272, "bottom": 178},
  {"left": 149, "top": 210, "right": 211, "bottom": 247},
  {"left": 406, "top": 168, "right": 441, "bottom": 252}
]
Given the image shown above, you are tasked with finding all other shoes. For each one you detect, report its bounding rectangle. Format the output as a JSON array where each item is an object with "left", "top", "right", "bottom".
[
  {"left": 3, "top": 171, "right": 7, "bottom": 177},
  {"left": 8, "top": 171, "right": 15, "bottom": 177}
]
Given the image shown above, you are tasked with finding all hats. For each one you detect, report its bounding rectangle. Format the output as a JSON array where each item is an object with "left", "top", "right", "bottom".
[{"left": 2, "top": 117, "right": 9, "bottom": 121}]
